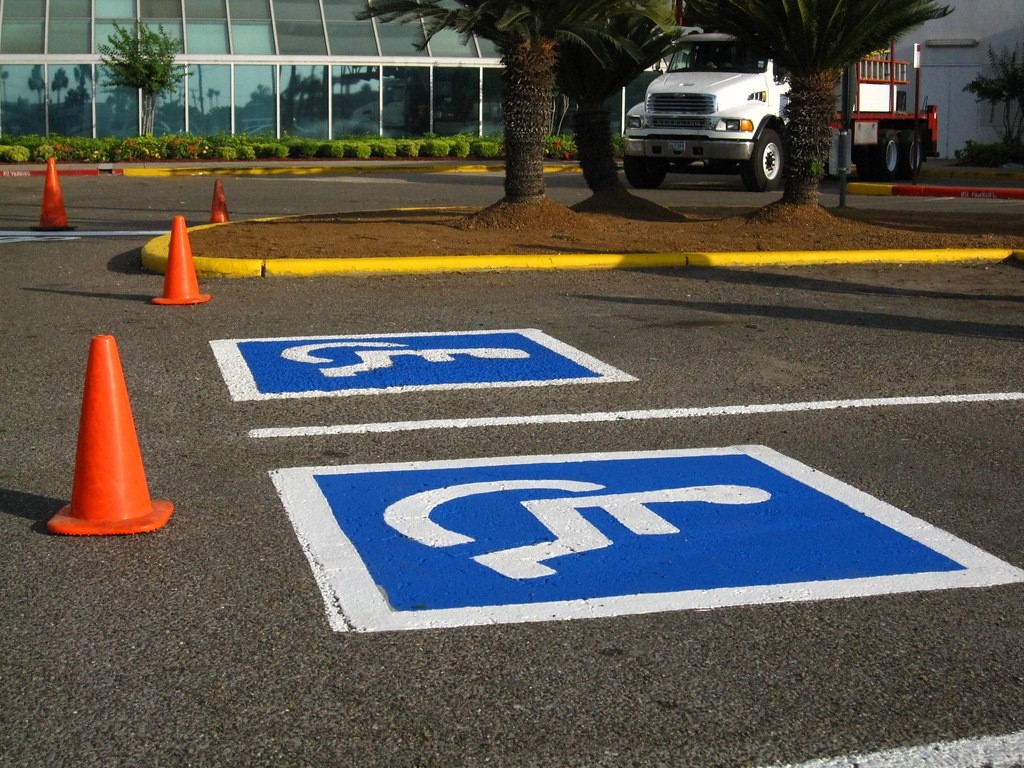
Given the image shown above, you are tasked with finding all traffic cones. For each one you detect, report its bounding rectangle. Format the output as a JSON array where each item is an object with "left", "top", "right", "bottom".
[
  {"left": 209, "top": 178, "right": 232, "bottom": 225},
  {"left": 152, "top": 215, "right": 212, "bottom": 308},
  {"left": 48, "top": 333, "right": 175, "bottom": 536},
  {"left": 31, "top": 155, "right": 78, "bottom": 234}
]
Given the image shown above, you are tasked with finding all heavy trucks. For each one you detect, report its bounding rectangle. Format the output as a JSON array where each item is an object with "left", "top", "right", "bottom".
[{"left": 623, "top": 27, "right": 942, "bottom": 195}]
